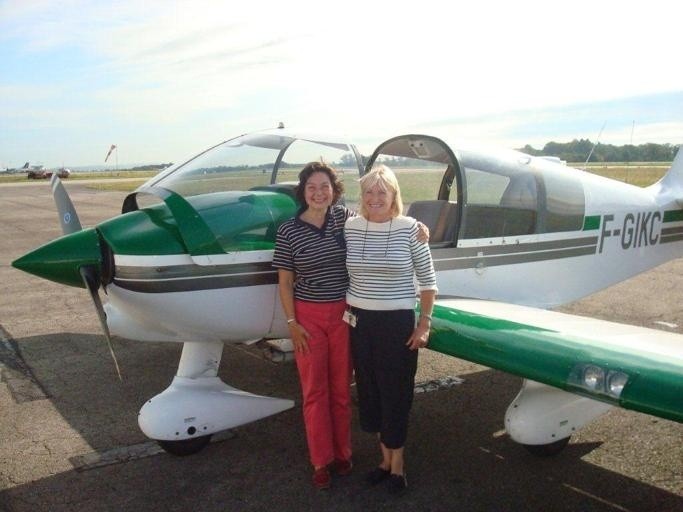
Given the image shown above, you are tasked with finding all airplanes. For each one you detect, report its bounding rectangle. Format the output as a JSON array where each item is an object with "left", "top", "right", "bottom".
[{"left": 10, "top": 125, "right": 683, "bottom": 455}]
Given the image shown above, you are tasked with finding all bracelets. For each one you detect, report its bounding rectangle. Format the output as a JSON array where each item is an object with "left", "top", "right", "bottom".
[
  {"left": 285, "top": 318, "right": 294, "bottom": 324},
  {"left": 417, "top": 313, "right": 433, "bottom": 322}
]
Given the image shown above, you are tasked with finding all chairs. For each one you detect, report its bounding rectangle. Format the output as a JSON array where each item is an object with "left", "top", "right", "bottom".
[{"left": 406, "top": 200, "right": 537, "bottom": 244}]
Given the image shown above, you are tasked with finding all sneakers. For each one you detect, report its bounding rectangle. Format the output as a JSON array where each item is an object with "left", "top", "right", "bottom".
[
  {"left": 363, "top": 467, "right": 389, "bottom": 484},
  {"left": 336, "top": 460, "right": 351, "bottom": 475},
  {"left": 314, "top": 467, "right": 331, "bottom": 488},
  {"left": 386, "top": 472, "right": 405, "bottom": 494}
]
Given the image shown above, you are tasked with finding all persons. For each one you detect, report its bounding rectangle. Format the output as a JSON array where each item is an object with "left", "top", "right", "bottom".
[
  {"left": 342, "top": 162, "right": 438, "bottom": 499},
  {"left": 270, "top": 156, "right": 430, "bottom": 491}
]
